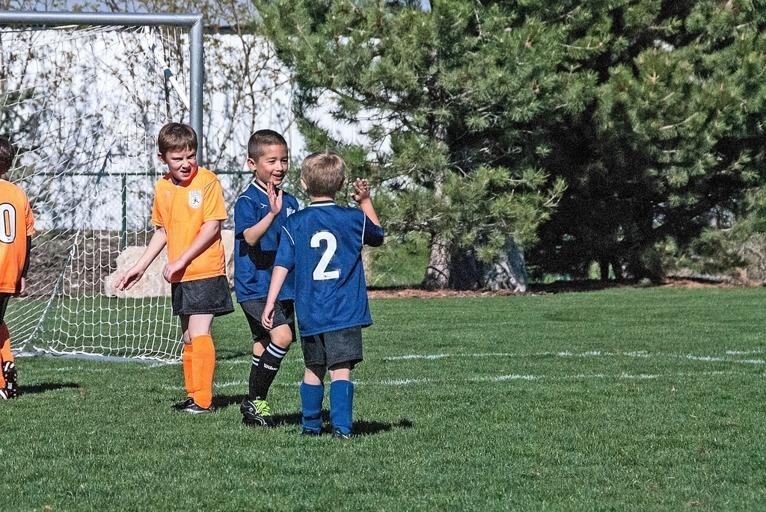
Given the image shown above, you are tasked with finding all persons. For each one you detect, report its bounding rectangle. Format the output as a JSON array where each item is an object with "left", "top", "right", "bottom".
[
  {"left": 261, "top": 151, "right": 385, "bottom": 436},
  {"left": 0, "top": 138, "right": 34, "bottom": 400},
  {"left": 233, "top": 129, "right": 300, "bottom": 425},
  {"left": 116, "top": 123, "right": 234, "bottom": 415}
]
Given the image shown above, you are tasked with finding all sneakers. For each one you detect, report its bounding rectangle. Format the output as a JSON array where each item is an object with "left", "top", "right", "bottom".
[
  {"left": 240, "top": 394, "right": 275, "bottom": 428},
  {"left": 172, "top": 397, "right": 215, "bottom": 415},
  {"left": 333, "top": 428, "right": 358, "bottom": 439},
  {"left": 0, "top": 360, "right": 18, "bottom": 400}
]
